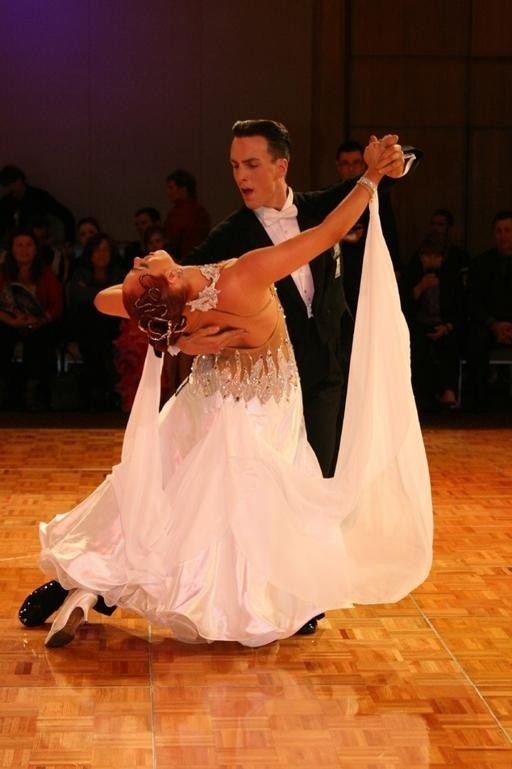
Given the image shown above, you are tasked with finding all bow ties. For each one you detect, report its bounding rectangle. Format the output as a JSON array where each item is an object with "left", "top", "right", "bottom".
[{"left": 262, "top": 204, "right": 298, "bottom": 227}]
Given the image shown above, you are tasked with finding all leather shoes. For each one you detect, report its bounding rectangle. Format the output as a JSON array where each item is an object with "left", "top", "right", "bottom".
[{"left": 19, "top": 580, "right": 66, "bottom": 626}]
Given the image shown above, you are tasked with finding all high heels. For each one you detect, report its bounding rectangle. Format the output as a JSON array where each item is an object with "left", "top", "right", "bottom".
[{"left": 45, "top": 588, "right": 98, "bottom": 647}]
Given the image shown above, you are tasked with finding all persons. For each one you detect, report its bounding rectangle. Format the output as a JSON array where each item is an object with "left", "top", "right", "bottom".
[
  {"left": 1, "top": 164, "right": 212, "bottom": 412},
  {"left": 320, "top": 140, "right": 511, "bottom": 414}
]
